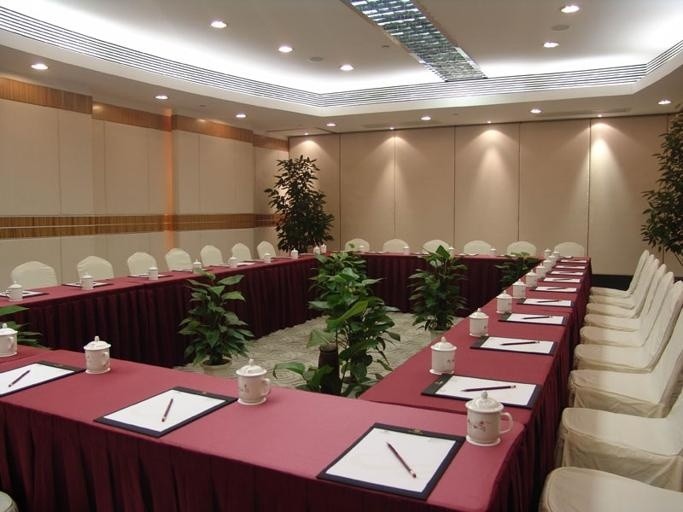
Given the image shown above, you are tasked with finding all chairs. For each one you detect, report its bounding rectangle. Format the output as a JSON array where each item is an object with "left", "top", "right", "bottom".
[
  {"left": 231, "top": 243, "right": 252, "bottom": 263},
  {"left": 77, "top": 256, "right": 114, "bottom": 281},
  {"left": 11, "top": 261, "right": 59, "bottom": 291},
  {"left": 200, "top": 245, "right": 223, "bottom": 266},
  {"left": 256, "top": 240, "right": 276, "bottom": 260},
  {"left": 463, "top": 240, "right": 492, "bottom": 256},
  {"left": 127, "top": 252, "right": 158, "bottom": 276},
  {"left": 506, "top": 241, "right": 536, "bottom": 257},
  {"left": 421, "top": 239, "right": 449, "bottom": 254},
  {"left": 344, "top": 238, "right": 369, "bottom": 252},
  {"left": 541, "top": 241, "right": 683, "bottom": 512},
  {"left": 164, "top": 248, "right": 193, "bottom": 272},
  {"left": 382, "top": 239, "right": 409, "bottom": 253}
]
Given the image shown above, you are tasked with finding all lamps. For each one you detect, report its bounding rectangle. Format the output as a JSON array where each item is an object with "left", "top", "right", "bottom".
[{"left": 339, "top": 0, "right": 488, "bottom": 82}]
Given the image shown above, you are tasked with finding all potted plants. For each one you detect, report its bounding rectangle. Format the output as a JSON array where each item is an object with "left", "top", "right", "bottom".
[
  {"left": 407, "top": 245, "right": 469, "bottom": 342},
  {"left": 178, "top": 268, "right": 255, "bottom": 377}
]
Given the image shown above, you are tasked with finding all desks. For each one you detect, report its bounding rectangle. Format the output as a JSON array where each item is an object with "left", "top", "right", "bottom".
[
  {"left": 358, "top": 257, "right": 592, "bottom": 512},
  {"left": 1, "top": 349, "right": 529, "bottom": 512},
  {"left": 0, "top": 252, "right": 334, "bottom": 369},
  {"left": 355, "top": 253, "right": 546, "bottom": 319}
]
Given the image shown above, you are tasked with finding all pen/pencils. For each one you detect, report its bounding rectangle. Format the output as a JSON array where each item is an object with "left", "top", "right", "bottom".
[
  {"left": 461, "top": 385, "right": 516, "bottom": 391},
  {"left": 553, "top": 278, "right": 571, "bottom": 280},
  {"left": 563, "top": 265, "right": 580, "bottom": 267},
  {"left": 387, "top": 442, "right": 416, "bottom": 478},
  {"left": 8, "top": 370, "right": 30, "bottom": 387},
  {"left": 5, "top": 291, "right": 31, "bottom": 294},
  {"left": 76, "top": 282, "right": 97, "bottom": 285},
  {"left": 567, "top": 259, "right": 584, "bottom": 261},
  {"left": 537, "top": 300, "right": 560, "bottom": 303},
  {"left": 560, "top": 271, "right": 576, "bottom": 273},
  {"left": 522, "top": 316, "right": 552, "bottom": 319},
  {"left": 547, "top": 287, "right": 568, "bottom": 290},
  {"left": 161, "top": 398, "right": 173, "bottom": 422},
  {"left": 501, "top": 341, "right": 540, "bottom": 345},
  {"left": 139, "top": 275, "right": 149, "bottom": 276}
]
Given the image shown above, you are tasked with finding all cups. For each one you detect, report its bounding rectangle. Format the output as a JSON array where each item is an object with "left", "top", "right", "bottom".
[
  {"left": 192, "top": 259, "right": 201, "bottom": 273},
  {"left": 235, "top": 360, "right": 270, "bottom": 404},
  {"left": 313, "top": 246, "right": 320, "bottom": 256},
  {"left": 548, "top": 255, "right": 557, "bottom": 266},
  {"left": 0, "top": 322, "right": 18, "bottom": 358},
  {"left": 448, "top": 246, "right": 455, "bottom": 256},
  {"left": 228, "top": 257, "right": 237, "bottom": 268},
  {"left": 496, "top": 290, "right": 513, "bottom": 314},
  {"left": 7, "top": 281, "right": 23, "bottom": 302},
  {"left": 84, "top": 337, "right": 111, "bottom": 375},
  {"left": 358, "top": 244, "right": 365, "bottom": 253},
  {"left": 535, "top": 264, "right": 546, "bottom": 279},
  {"left": 543, "top": 248, "right": 551, "bottom": 259},
  {"left": 402, "top": 245, "right": 410, "bottom": 255},
  {"left": 489, "top": 248, "right": 497, "bottom": 257},
  {"left": 291, "top": 248, "right": 298, "bottom": 259},
  {"left": 552, "top": 251, "right": 561, "bottom": 262},
  {"left": 512, "top": 279, "right": 526, "bottom": 297},
  {"left": 80, "top": 272, "right": 94, "bottom": 290},
  {"left": 263, "top": 253, "right": 271, "bottom": 264},
  {"left": 469, "top": 308, "right": 489, "bottom": 337},
  {"left": 321, "top": 244, "right": 326, "bottom": 253},
  {"left": 543, "top": 259, "right": 552, "bottom": 272},
  {"left": 466, "top": 392, "right": 512, "bottom": 446},
  {"left": 148, "top": 266, "right": 158, "bottom": 280},
  {"left": 525, "top": 270, "right": 538, "bottom": 288},
  {"left": 429, "top": 337, "right": 457, "bottom": 376}
]
